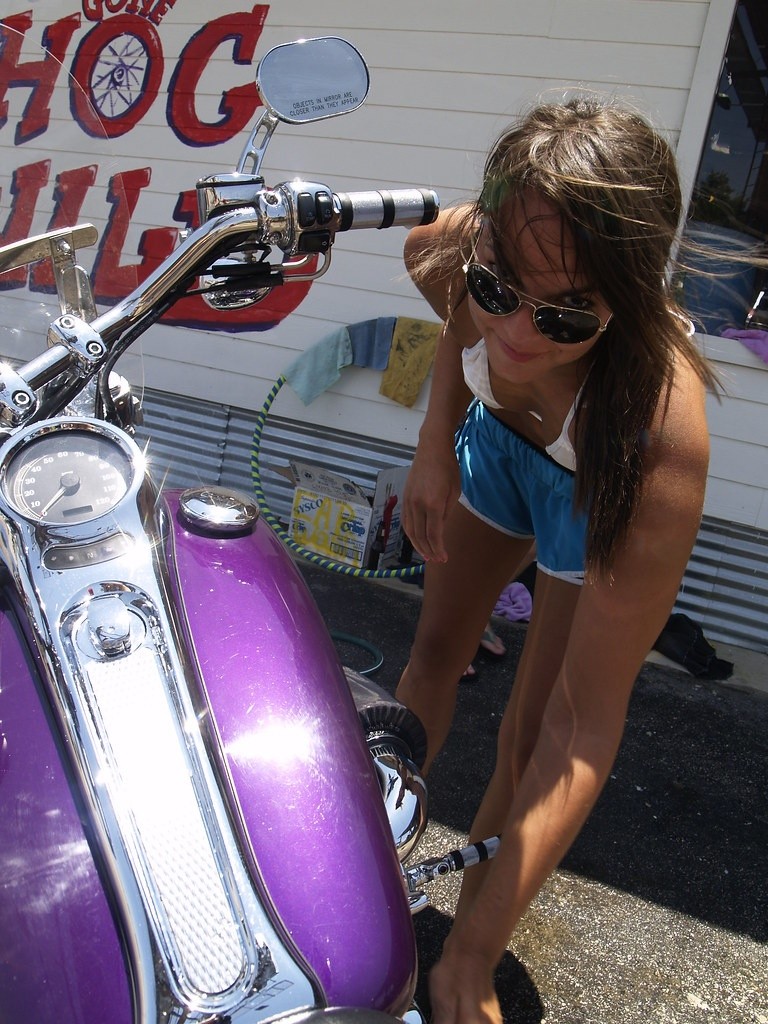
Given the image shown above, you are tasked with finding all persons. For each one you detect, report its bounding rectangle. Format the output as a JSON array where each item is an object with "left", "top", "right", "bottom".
[{"left": 401, "top": 100, "right": 725, "bottom": 1024}]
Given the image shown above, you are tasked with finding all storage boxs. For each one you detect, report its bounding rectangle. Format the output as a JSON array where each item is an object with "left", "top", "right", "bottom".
[{"left": 266, "top": 459, "right": 411, "bottom": 570}]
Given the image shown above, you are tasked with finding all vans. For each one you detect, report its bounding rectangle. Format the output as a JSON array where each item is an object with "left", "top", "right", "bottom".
[{"left": 0, "top": 1, "right": 768, "bottom": 724}]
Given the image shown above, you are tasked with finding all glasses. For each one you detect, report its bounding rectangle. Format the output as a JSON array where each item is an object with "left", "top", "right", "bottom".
[{"left": 463, "top": 229, "right": 614, "bottom": 346}]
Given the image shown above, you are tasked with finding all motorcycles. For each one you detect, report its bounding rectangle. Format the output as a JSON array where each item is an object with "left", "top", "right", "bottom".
[{"left": 0, "top": 35, "right": 516, "bottom": 1024}]
